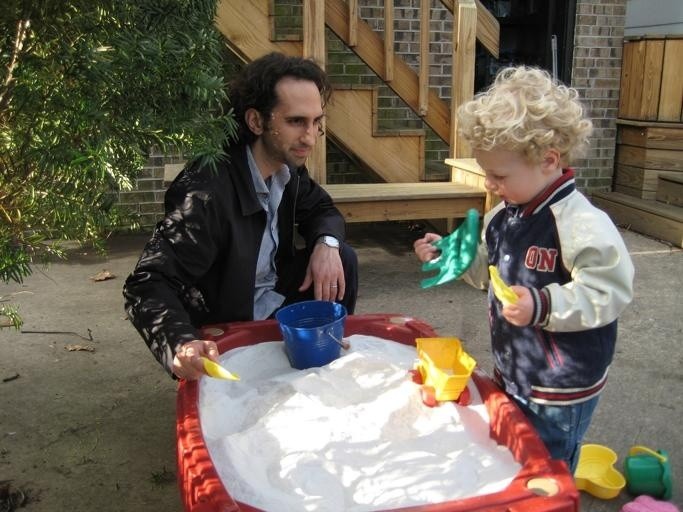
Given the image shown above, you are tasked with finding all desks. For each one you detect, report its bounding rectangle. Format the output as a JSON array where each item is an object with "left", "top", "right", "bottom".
[
  {"left": 316, "top": 181, "right": 486, "bottom": 234},
  {"left": 445, "top": 158, "right": 498, "bottom": 211}
]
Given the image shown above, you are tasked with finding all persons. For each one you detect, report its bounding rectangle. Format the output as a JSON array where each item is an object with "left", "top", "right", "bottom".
[
  {"left": 119, "top": 50, "right": 359, "bottom": 384},
  {"left": 410, "top": 63, "right": 637, "bottom": 474}
]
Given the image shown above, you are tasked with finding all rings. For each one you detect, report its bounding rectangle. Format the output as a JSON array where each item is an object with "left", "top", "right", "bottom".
[{"left": 330, "top": 285, "right": 338, "bottom": 289}]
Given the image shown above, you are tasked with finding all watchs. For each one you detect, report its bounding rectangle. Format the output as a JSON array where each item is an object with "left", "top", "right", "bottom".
[{"left": 315, "top": 235, "right": 340, "bottom": 250}]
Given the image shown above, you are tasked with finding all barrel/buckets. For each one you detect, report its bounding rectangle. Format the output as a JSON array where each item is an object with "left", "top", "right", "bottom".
[
  {"left": 276, "top": 299, "right": 351, "bottom": 370},
  {"left": 624, "top": 445, "right": 673, "bottom": 499}
]
